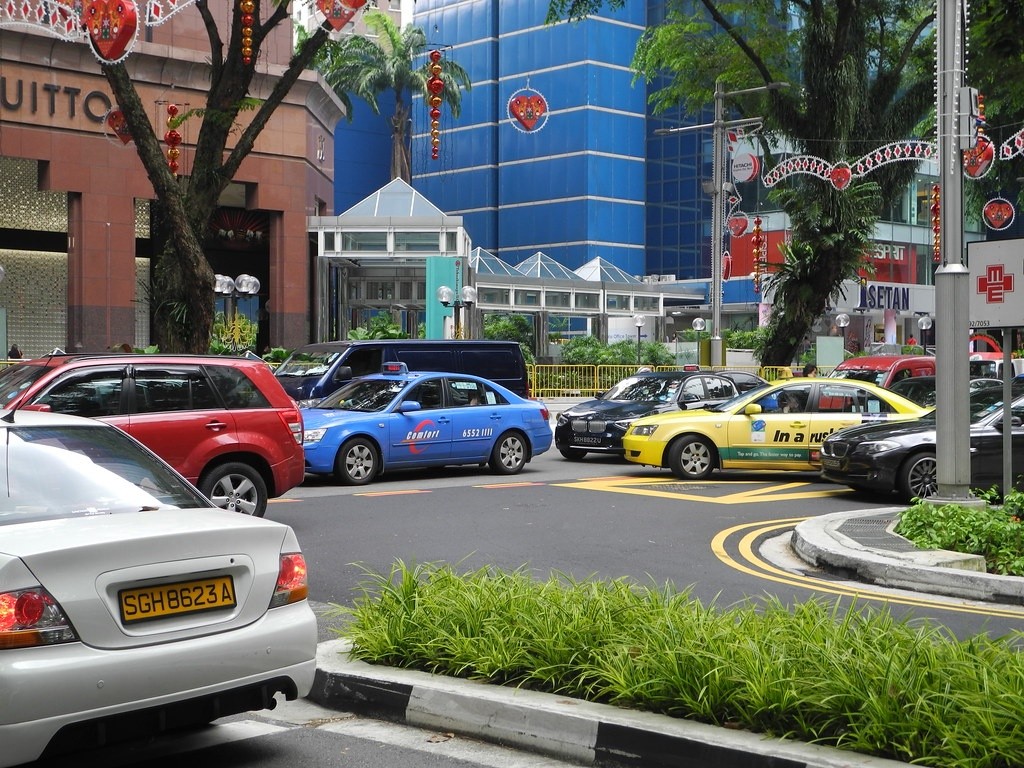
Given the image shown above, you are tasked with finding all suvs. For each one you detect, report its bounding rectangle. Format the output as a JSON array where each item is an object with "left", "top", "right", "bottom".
[{"left": 1, "top": 354, "right": 305, "bottom": 519}]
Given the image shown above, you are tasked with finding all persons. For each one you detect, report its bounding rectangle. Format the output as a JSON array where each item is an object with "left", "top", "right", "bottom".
[
  {"left": 8, "top": 344, "right": 22, "bottom": 366},
  {"left": 802, "top": 336, "right": 811, "bottom": 352},
  {"left": 907, "top": 334, "right": 917, "bottom": 345},
  {"left": 879, "top": 337, "right": 885, "bottom": 343},
  {"left": 802, "top": 364, "right": 817, "bottom": 378}
]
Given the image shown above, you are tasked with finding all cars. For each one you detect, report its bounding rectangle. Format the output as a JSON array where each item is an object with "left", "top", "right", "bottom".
[
  {"left": 299, "top": 362, "right": 555, "bottom": 486},
  {"left": 555, "top": 355, "right": 1024, "bottom": 503},
  {"left": 0, "top": 409, "right": 318, "bottom": 767}
]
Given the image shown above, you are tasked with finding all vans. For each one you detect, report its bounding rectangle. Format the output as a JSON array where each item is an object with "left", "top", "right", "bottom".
[{"left": 274, "top": 339, "right": 528, "bottom": 410}]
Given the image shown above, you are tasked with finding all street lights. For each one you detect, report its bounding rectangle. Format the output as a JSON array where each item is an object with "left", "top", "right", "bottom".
[
  {"left": 214, "top": 274, "right": 260, "bottom": 355},
  {"left": 835, "top": 314, "right": 850, "bottom": 348},
  {"left": 692, "top": 318, "right": 705, "bottom": 364},
  {"left": 918, "top": 317, "right": 932, "bottom": 356},
  {"left": 634, "top": 314, "right": 647, "bottom": 365},
  {"left": 438, "top": 286, "right": 477, "bottom": 339},
  {"left": 654, "top": 78, "right": 790, "bottom": 364}
]
